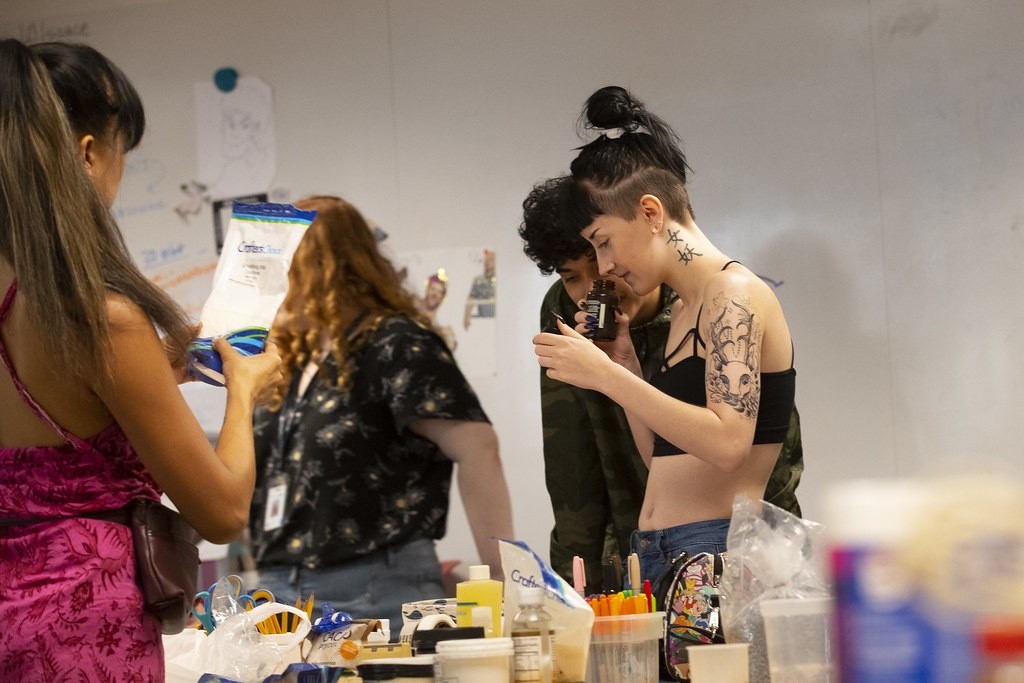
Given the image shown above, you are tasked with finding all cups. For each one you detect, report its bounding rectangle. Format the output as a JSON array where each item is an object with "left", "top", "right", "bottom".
[
  {"left": 587, "top": 611, "right": 666, "bottom": 683},
  {"left": 759, "top": 597, "right": 843, "bottom": 683},
  {"left": 686, "top": 643, "right": 750, "bottom": 682}
]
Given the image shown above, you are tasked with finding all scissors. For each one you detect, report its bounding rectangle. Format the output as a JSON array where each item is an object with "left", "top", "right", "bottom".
[{"left": 191, "top": 575, "right": 276, "bottom": 634}]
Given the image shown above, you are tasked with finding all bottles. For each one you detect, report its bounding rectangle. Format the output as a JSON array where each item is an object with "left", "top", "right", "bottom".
[
  {"left": 509, "top": 588, "right": 555, "bottom": 683},
  {"left": 586, "top": 278, "right": 618, "bottom": 342},
  {"left": 456, "top": 565, "right": 503, "bottom": 638},
  {"left": 436, "top": 638, "right": 514, "bottom": 683}
]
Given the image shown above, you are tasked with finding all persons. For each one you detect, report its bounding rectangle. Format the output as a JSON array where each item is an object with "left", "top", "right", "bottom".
[
  {"left": 534, "top": 87, "right": 794, "bottom": 683},
  {"left": 0, "top": 39, "right": 285, "bottom": 683},
  {"left": 225, "top": 196, "right": 516, "bottom": 646},
  {"left": 520, "top": 175, "right": 804, "bottom": 599},
  {"left": 414, "top": 276, "right": 446, "bottom": 321},
  {"left": 463, "top": 251, "right": 496, "bottom": 329}
]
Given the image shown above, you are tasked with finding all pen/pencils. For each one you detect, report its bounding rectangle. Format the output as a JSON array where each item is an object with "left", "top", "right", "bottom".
[
  {"left": 572, "top": 556, "right": 586, "bottom": 599},
  {"left": 585, "top": 580, "right": 656, "bottom": 683},
  {"left": 627, "top": 553, "right": 641, "bottom": 595},
  {"left": 256, "top": 589, "right": 315, "bottom": 635}
]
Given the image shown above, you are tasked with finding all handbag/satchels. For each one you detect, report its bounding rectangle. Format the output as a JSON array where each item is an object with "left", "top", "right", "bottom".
[
  {"left": 159, "top": 602, "right": 313, "bottom": 683},
  {"left": 657, "top": 548, "right": 739, "bottom": 683},
  {"left": 4, "top": 496, "right": 202, "bottom": 635}
]
